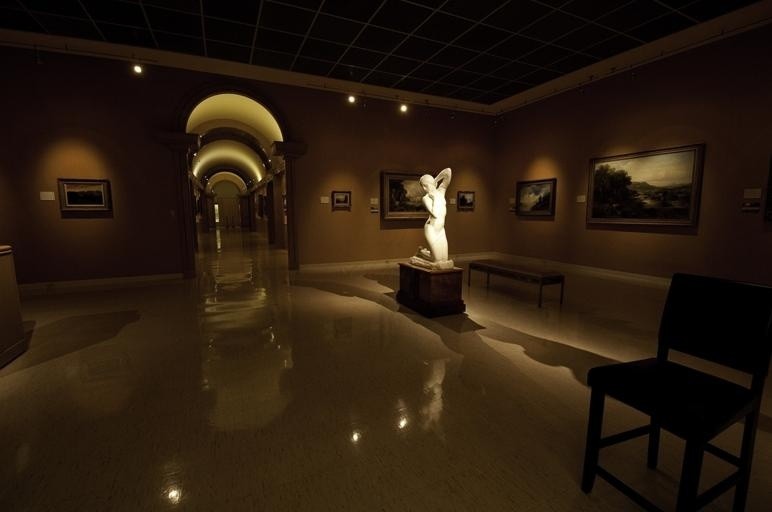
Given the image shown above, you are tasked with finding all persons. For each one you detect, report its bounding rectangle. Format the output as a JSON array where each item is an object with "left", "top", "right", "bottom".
[{"left": 416, "top": 168, "right": 452, "bottom": 263}]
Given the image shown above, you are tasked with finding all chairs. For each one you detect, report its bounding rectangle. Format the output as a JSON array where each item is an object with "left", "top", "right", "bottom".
[{"left": 579, "top": 273, "right": 770, "bottom": 510}]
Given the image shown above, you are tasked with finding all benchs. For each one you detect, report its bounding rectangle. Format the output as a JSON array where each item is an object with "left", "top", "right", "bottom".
[{"left": 467, "top": 259, "right": 566, "bottom": 308}]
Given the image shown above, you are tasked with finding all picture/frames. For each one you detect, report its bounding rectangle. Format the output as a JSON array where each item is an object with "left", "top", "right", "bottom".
[
  {"left": 331, "top": 191, "right": 351, "bottom": 208},
  {"left": 381, "top": 170, "right": 441, "bottom": 222},
  {"left": 56, "top": 177, "right": 113, "bottom": 217},
  {"left": 514, "top": 177, "right": 557, "bottom": 217},
  {"left": 584, "top": 143, "right": 707, "bottom": 228},
  {"left": 458, "top": 191, "right": 475, "bottom": 209}
]
